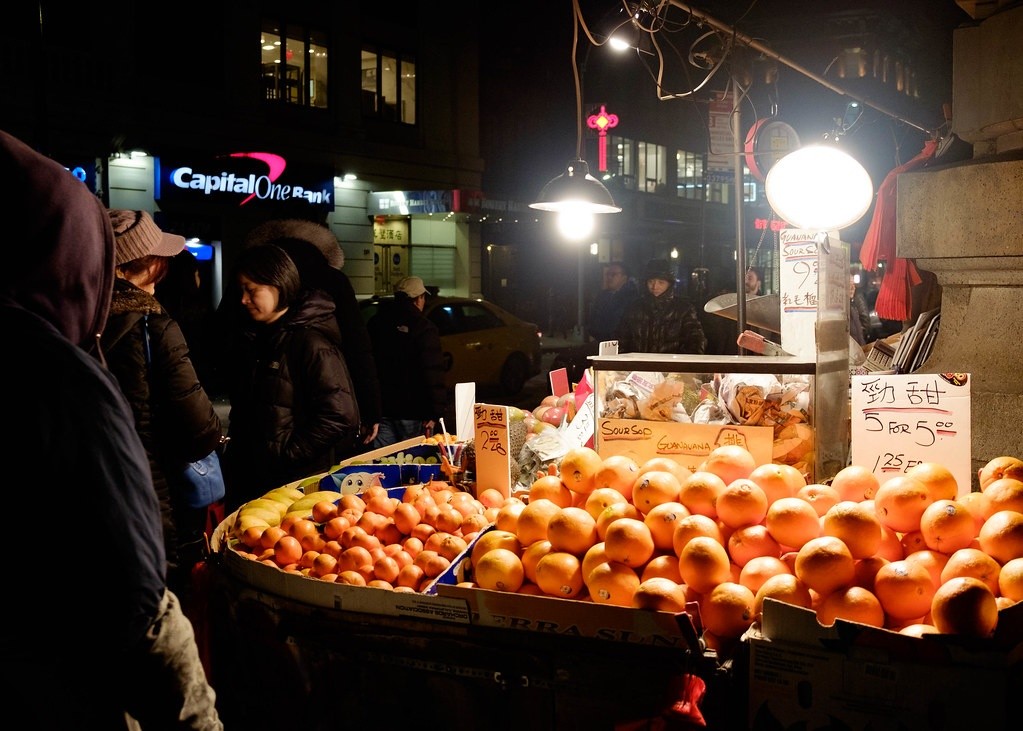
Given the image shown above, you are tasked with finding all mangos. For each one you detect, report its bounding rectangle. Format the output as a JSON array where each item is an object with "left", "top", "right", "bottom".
[
  {"left": 234, "top": 488, "right": 346, "bottom": 538},
  {"left": 507, "top": 392, "right": 575, "bottom": 440}
]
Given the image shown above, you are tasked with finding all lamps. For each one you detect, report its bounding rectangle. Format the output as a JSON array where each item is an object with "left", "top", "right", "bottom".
[
  {"left": 525, "top": 0, "right": 624, "bottom": 246},
  {"left": 762, "top": 103, "right": 884, "bottom": 236}
]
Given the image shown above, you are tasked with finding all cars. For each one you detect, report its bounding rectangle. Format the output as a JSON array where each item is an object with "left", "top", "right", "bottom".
[{"left": 360, "top": 296, "right": 543, "bottom": 397}]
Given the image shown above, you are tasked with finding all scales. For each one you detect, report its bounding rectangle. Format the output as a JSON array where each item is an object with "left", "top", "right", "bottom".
[{"left": 702, "top": 67, "right": 805, "bottom": 335}]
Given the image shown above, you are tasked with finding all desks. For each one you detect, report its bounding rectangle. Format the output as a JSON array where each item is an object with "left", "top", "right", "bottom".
[{"left": 265, "top": 62, "right": 300, "bottom": 105}]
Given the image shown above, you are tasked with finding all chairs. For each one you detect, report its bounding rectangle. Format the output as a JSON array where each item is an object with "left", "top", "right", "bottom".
[{"left": 263, "top": 62, "right": 303, "bottom": 105}]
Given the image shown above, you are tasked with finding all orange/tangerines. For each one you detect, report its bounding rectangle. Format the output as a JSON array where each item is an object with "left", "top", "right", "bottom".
[
  {"left": 419, "top": 433, "right": 457, "bottom": 444},
  {"left": 279, "top": 482, "right": 505, "bottom": 593},
  {"left": 469, "top": 444, "right": 1023, "bottom": 652},
  {"left": 232, "top": 526, "right": 286, "bottom": 568}
]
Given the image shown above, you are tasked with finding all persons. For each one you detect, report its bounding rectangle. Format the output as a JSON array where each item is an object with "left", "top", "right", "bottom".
[
  {"left": 0, "top": 130, "right": 221, "bottom": 731},
  {"left": 105, "top": 205, "right": 451, "bottom": 688},
  {"left": 585, "top": 259, "right": 872, "bottom": 356}
]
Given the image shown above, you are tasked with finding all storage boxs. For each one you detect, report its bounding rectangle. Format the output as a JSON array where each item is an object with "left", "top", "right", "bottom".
[{"left": 585, "top": 351, "right": 852, "bottom": 486}]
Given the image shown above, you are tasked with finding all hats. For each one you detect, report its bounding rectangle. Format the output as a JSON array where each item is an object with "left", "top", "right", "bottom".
[
  {"left": 644, "top": 259, "right": 672, "bottom": 282},
  {"left": 396, "top": 275, "right": 431, "bottom": 297},
  {"left": 107, "top": 208, "right": 186, "bottom": 266}
]
